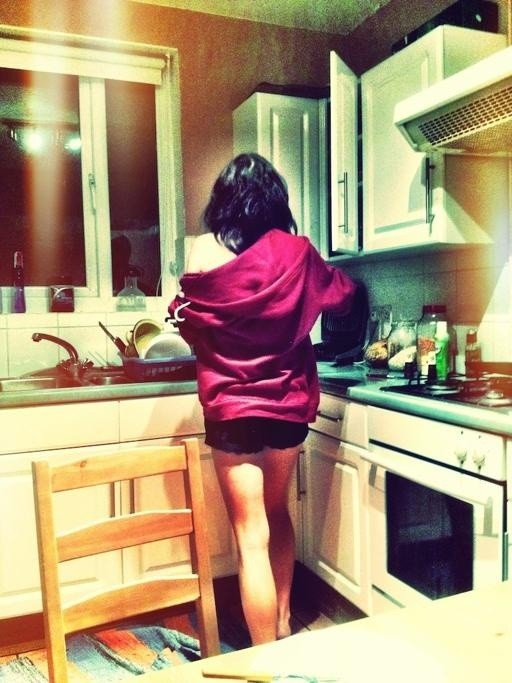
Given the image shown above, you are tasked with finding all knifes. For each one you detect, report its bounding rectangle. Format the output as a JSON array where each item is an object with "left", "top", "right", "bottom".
[{"left": 98, "top": 322, "right": 126, "bottom": 355}]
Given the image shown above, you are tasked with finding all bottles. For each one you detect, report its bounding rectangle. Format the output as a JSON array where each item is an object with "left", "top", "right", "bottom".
[
  {"left": 465, "top": 329, "right": 482, "bottom": 378},
  {"left": 116, "top": 270, "right": 147, "bottom": 312},
  {"left": 11, "top": 251, "right": 26, "bottom": 313},
  {"left": 388, "top": 304, "right": 457, "bottom": 379}
]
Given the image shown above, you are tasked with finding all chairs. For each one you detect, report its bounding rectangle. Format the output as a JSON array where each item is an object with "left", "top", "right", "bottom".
[{"left": 0, "top": 437, "right": 220, "bottom": 682}]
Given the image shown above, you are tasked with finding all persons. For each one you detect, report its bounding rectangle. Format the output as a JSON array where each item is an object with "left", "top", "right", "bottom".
[{"left": 164, "top": 151, "right": 357, "bottom": 648}]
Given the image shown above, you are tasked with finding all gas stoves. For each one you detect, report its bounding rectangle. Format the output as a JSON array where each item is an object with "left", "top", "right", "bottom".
[{"left": 379, "top": 375, "right": 512, "bottom": 408}]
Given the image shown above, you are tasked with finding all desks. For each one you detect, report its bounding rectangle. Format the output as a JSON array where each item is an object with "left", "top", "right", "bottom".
[{"left": 128, "top": 575, "right": 512, "bottom": 681}]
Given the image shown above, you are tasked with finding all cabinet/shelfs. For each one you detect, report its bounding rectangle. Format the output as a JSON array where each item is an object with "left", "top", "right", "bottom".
[
  {"left": 320, "top": 22, "right": 505, "bottom": 264},
  {"left": 295, "top": 390, "right": 369, "bottom": 615},
  {"left": 231, "top": 91, "right": 319, "bottom": 262},
  {"left": 1, "top": 399, "right": 118, "bottom": 623},
  {"left": 119, "top": 393, "right": 294, "bottom": 585}
]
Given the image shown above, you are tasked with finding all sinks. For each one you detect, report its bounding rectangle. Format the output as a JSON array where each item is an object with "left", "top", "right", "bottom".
[
  {"left": 88, "top": 372, "right": 130, "bottom": 384},
  {"left": 1, "top": 378, "right": 82, "bottom": 391}
]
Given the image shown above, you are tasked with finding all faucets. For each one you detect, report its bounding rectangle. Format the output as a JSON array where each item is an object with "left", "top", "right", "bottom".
[{"left": 33, "top": 332, "right": 78, "bottom": 361}]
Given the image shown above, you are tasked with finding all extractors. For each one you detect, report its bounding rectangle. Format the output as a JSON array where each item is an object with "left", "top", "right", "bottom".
[{"left": 392, "top": 45, "right": 511, "bottom": 162}]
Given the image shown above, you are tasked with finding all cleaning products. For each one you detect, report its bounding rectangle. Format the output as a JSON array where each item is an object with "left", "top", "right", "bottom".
[{"left": 14, "top": 251, "right": 25, "bottom": 313}]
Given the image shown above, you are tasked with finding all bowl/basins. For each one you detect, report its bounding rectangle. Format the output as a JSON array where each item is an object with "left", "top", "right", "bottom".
[{"left": 126, "top": 320, "right": 192, "bottom": 359}]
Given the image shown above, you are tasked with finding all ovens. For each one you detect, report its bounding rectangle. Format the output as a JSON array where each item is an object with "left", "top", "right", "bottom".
[{"left": 360, "top": 405, "right": 505, "bottom": 607}]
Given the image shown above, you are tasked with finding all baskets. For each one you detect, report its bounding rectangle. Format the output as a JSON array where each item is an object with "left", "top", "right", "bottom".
[{"left": 118, "top": 352, "right": 198, "bottom": 381}]
